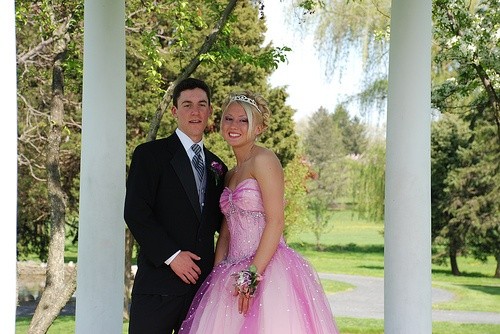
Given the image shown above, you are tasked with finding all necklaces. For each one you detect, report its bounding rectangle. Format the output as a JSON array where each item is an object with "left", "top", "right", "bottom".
[{"left": 233, "top": 144, "right": 255, "bottom": 174}]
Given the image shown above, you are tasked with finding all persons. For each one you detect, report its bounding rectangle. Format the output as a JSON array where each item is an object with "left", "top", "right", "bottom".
[
  {"left": 123, "top": 78, "right": 229, "bottom": 334},
  {"left": 179, "top": 91, "right": 339, "bottom": 334}
]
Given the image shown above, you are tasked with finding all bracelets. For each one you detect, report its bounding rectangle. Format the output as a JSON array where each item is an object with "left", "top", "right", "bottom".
[{"left": 229, "top": 264, "right": 263, "bottom": 297}]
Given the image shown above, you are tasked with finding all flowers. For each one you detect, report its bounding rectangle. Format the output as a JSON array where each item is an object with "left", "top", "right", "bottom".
[{"left": 211, "top": 161, "right": 224, "bottom": 185}]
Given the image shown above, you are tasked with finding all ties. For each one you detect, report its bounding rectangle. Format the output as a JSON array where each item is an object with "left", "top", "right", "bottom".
[{"left": 191, "top": 144, "right": 205, "bottom": 212}]
